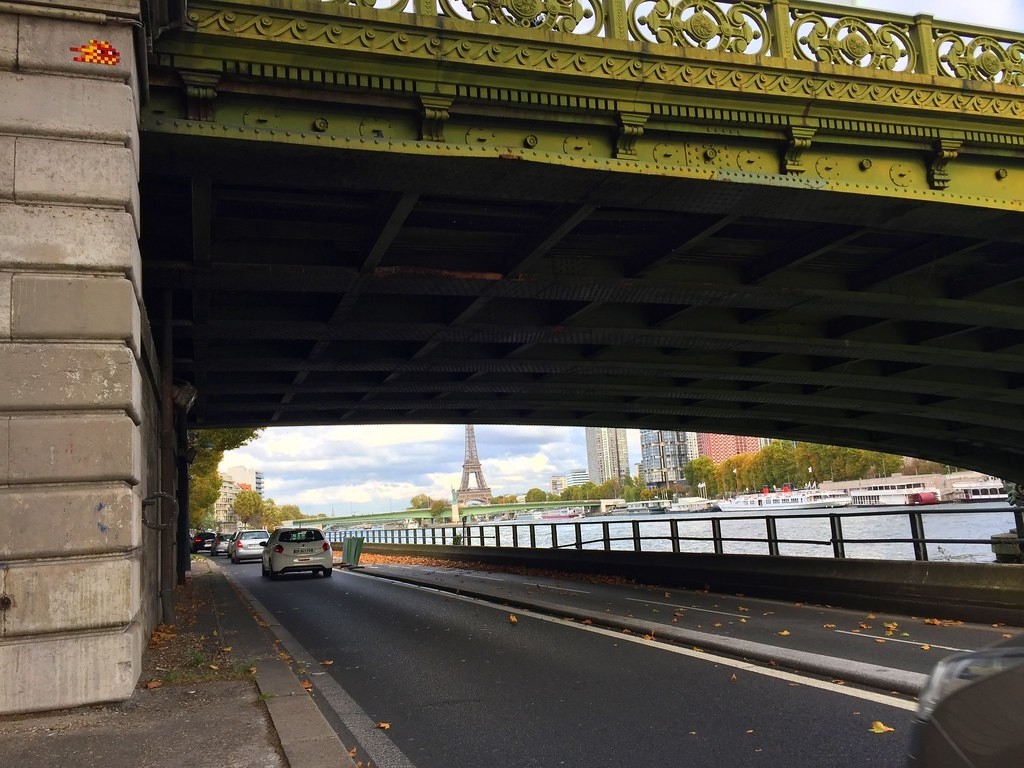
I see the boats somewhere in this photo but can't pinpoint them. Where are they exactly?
[493,507,587,520]
[611,482,956,515]
[955,476,1010,502]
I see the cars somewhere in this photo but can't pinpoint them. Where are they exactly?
[259,526,333,582]
[211,533,234,556]
[227,530,271,564]
[190,531,216,553]
